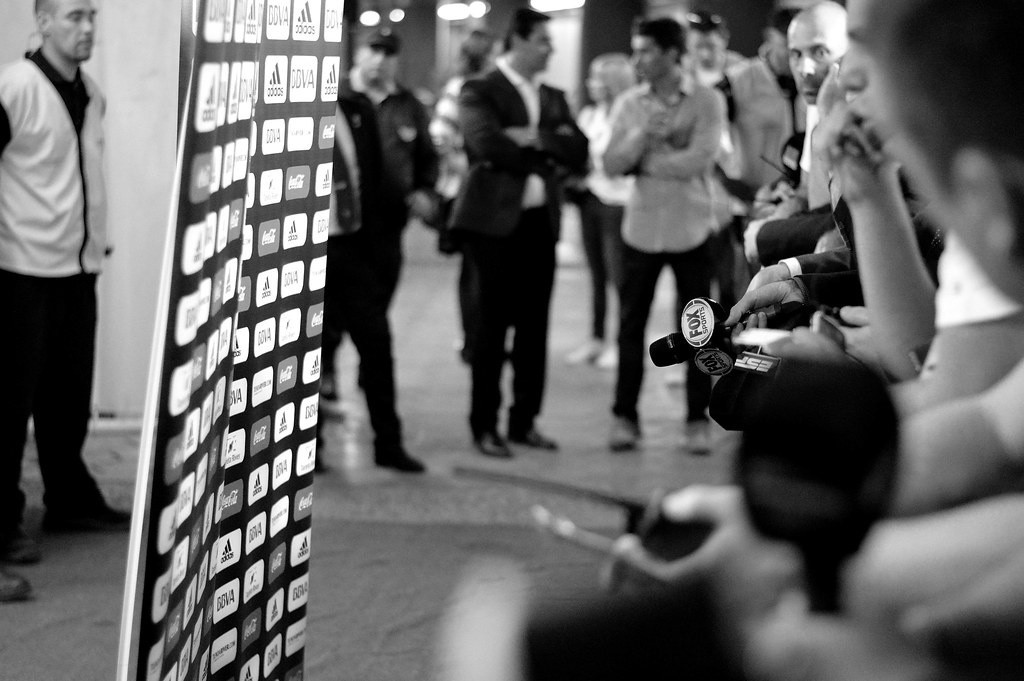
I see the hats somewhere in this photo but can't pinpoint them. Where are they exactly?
[363,30,403,49]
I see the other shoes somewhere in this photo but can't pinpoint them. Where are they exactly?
[374,441,426,476]
[683,414,714,451]
[608,410,638,447]
[595,346,618,368]
[567,338,602,362]
[0,529,39,567]
[41,507,131,534]
[0,570,32,602]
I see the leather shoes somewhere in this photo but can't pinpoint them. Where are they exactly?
[507,425,554,448]
[472,426,512,457]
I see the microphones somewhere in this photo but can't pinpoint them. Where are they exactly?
[650,296,806,376]
[709,344,781,432]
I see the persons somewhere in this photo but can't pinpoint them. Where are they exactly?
[0,0,133,604]
[316,0,1024,681]
